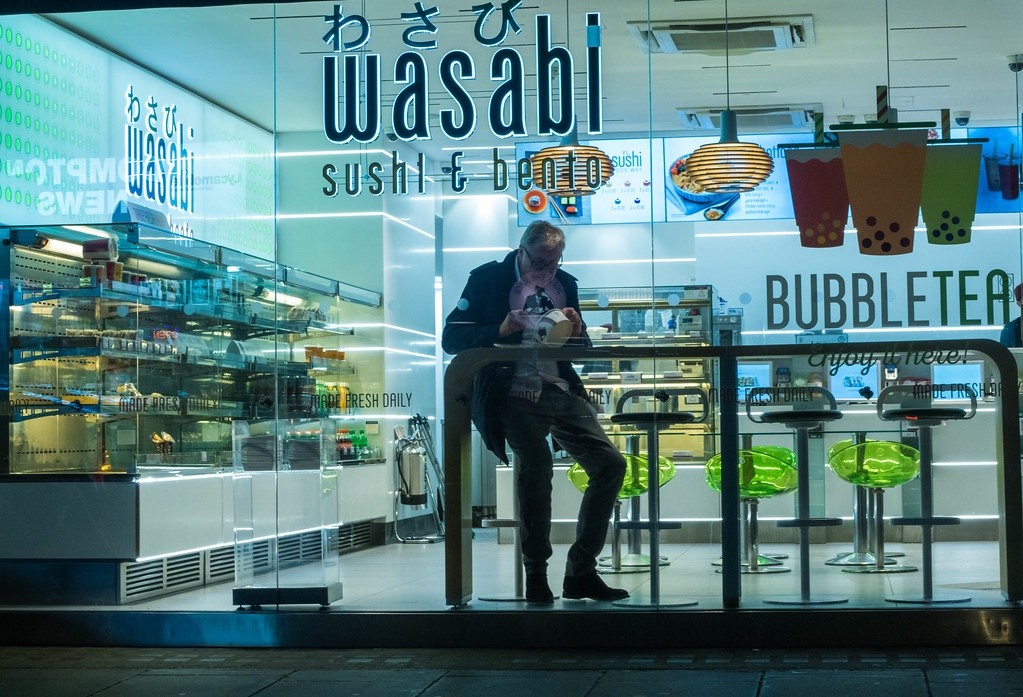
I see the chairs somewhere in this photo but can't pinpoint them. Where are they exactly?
[829,439,878,459]
[633,454,676,565]
[706,451,790,574]
[829,441,920,574]
[571,454,650,575]
[751,445,796,468]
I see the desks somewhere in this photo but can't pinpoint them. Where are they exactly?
[446,340,1023,606]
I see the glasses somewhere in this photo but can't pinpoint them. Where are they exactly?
[1015,294,1023,301]
[520,244,564,272]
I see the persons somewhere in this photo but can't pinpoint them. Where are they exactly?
[443,221,632,604]
[999,283,1023,348]
[522,284,555,316]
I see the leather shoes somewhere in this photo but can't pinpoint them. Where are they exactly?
[562,571,630,602]
[526,572,554,605]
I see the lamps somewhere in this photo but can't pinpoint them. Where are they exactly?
[684,1,775,193]
[531,0,615,197]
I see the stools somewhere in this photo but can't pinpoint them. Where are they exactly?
[876,384,977,603]
[746,386,848,604]
[612,387,709,608]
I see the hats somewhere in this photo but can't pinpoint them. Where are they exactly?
[1014,282,1022,296]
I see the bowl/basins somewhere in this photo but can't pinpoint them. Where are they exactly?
[532,307,574,348]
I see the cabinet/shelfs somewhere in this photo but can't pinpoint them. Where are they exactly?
[1,222,382,481]
[578,286,715,460]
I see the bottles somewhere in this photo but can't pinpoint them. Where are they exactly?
[82,236,149,290]
[286,426,381,462]
